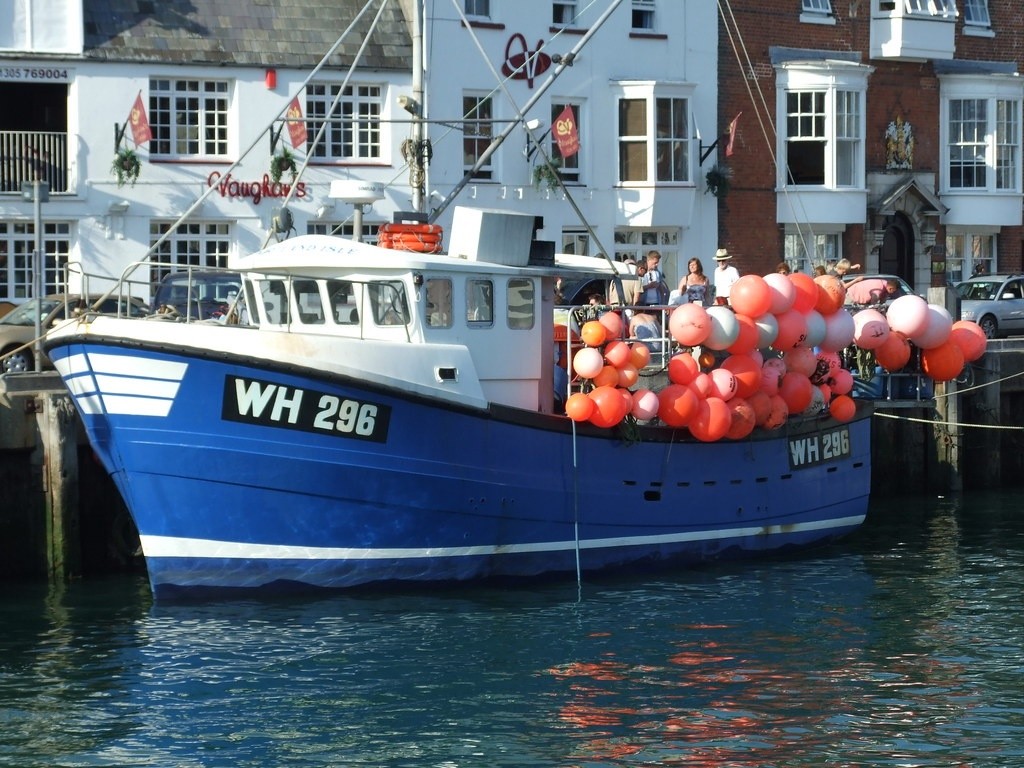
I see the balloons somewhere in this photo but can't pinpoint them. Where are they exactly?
[730,273,856,430]
[853,295,987,382]
[660,303,762,442]
[565,311,659,429]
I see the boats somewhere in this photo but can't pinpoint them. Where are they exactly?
[41,0,875,602]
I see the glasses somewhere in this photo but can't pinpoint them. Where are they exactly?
[717,260,726,262]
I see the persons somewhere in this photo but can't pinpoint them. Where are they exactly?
[757,258,863,289]
[970,264,985,276]
[588,294,606,321]
[629,300,664,365]
[610,250,667,306]
[678,257,715,305]
[843,278,901,311]
[712,249,740,305]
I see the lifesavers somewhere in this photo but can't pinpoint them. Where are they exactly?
[378,224,443,253]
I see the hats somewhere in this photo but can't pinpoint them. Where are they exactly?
[594,253,605,258]
[624,258,636,265]
[712,248,733,260]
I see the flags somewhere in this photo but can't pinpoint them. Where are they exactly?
[550,104,579,159]
[286,94,308,149]
[726,117,737,156]
[128,94,152,149]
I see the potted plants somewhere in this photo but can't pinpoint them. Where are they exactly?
[533,158,564,197]
[270,146,299,185]
[109,146,141,189]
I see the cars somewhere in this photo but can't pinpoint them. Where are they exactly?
[843,271,926,315]
[0,294,146,374]
[954,274,1023,339]
[146,269,245,322]
